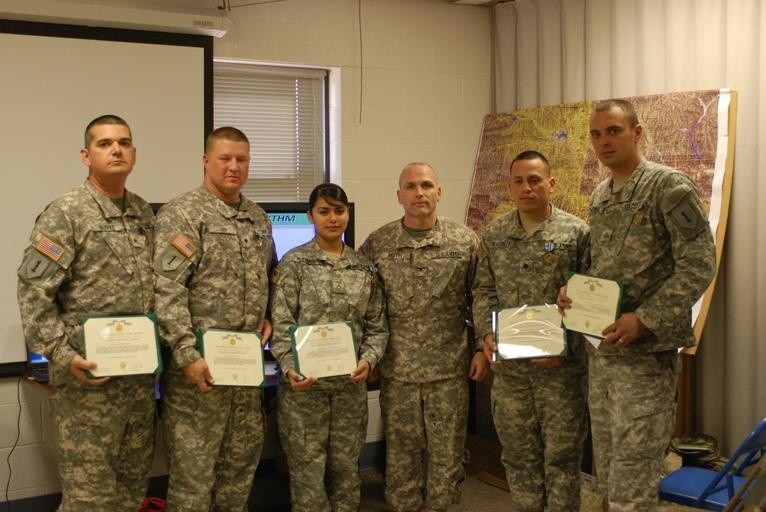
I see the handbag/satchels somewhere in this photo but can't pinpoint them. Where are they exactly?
[668,433,722,469]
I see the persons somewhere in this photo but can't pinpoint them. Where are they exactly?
[473,151,589,512]
[270,182,389,512]
[16,113,153,512]
[153,126,278,512]
[358,162,490,512]
[556,99,717,512]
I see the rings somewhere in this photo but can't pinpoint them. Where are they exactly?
[618,339,625,345]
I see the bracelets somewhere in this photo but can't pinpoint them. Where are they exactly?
[475,348,484,352]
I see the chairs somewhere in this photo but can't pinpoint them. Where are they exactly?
[658,418,766,511]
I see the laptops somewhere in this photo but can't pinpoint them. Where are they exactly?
[27,336,49,383]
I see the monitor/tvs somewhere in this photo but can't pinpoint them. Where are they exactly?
[150,203,355,361]
